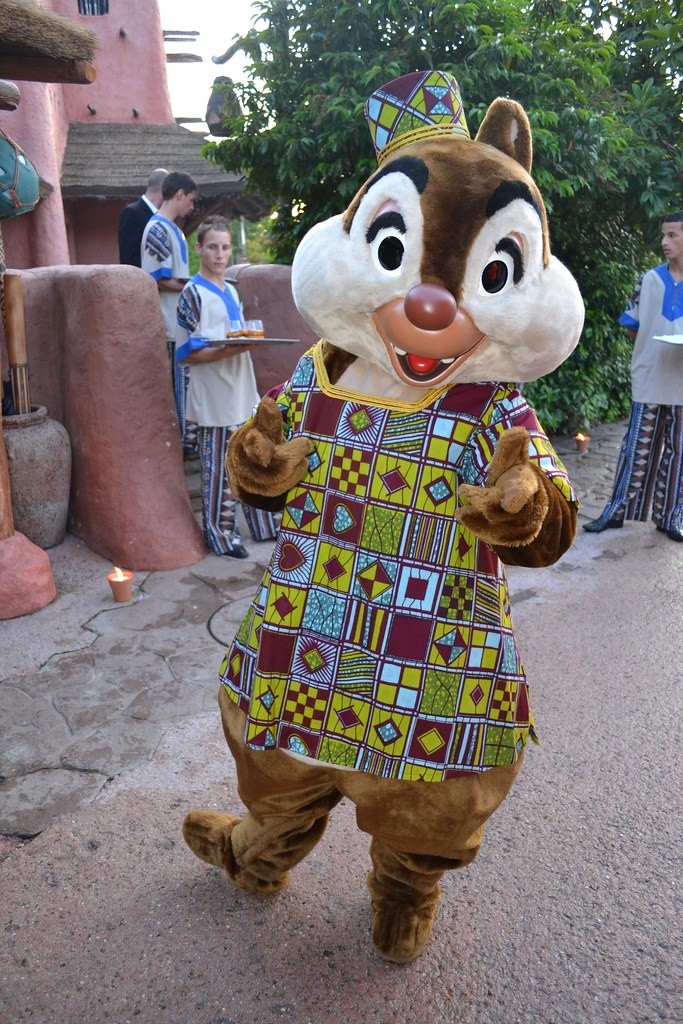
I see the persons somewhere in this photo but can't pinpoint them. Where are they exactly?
[138,172,201,459]
[582,212,683,543]
[120,169,171,268]
[176,215,284,560]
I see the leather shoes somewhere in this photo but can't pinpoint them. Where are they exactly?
[583,514,623,534]
[657,525,683,542]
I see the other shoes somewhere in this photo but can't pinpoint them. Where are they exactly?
[224,546,249,558]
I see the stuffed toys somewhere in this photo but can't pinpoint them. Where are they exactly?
[183,70,584,963]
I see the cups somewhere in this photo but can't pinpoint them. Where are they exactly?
[107,572,134,602]
[224,319,265,339]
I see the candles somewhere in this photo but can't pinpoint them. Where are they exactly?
[106,564,134,604]
[575,432,591,451]
